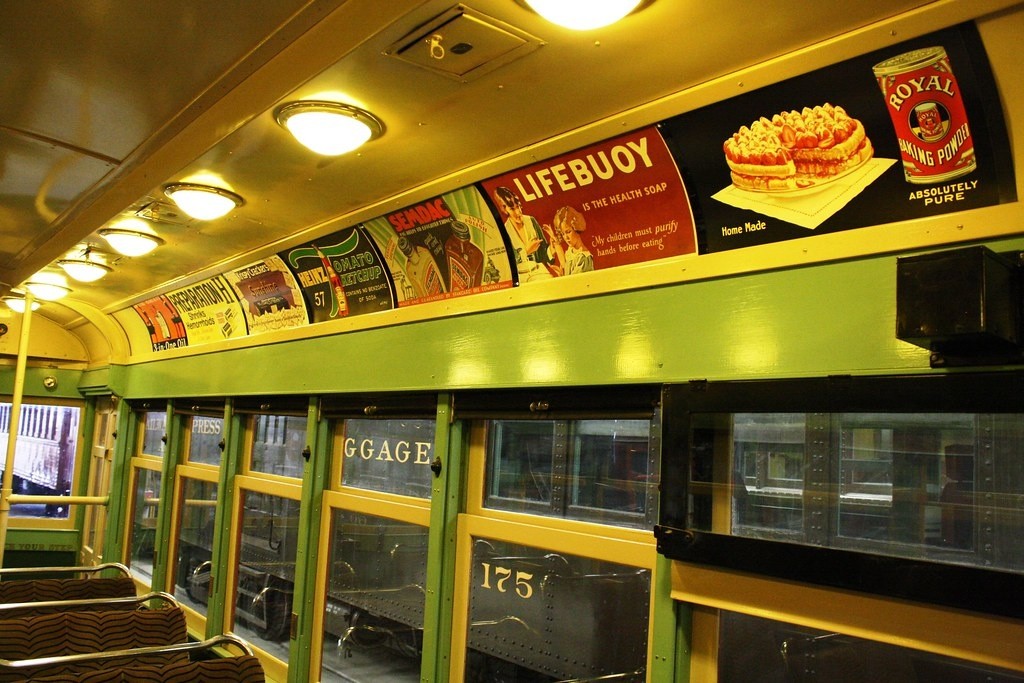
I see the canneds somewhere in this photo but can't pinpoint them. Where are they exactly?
[915,103,944,141]
[871,46,977,185]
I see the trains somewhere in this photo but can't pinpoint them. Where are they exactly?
[0,373,1024,683]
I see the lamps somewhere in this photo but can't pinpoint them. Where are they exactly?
[3,298,44,315]
[164,184,245,221]
[514,0,656,31]
[278,100,385,157]
[25,282,73,300]
[99,230,165,257]
[59,260,114,283]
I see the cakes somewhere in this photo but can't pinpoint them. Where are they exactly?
[724,102,873,193]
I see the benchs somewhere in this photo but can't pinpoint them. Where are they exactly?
[0,562,265,683]
